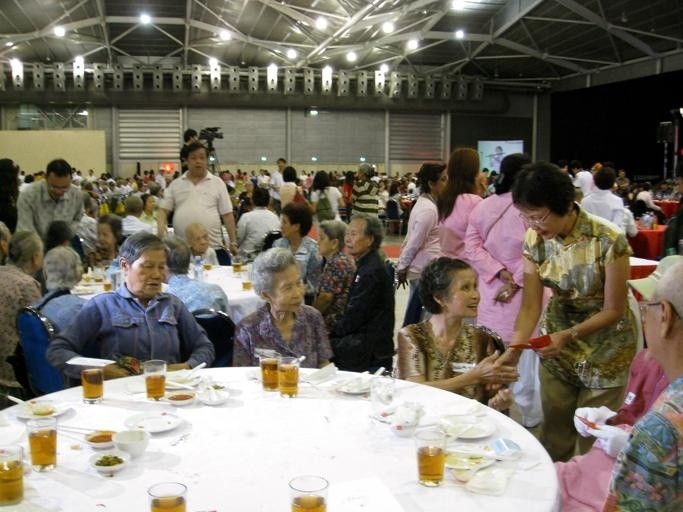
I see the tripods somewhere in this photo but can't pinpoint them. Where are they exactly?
[206,147,223,176]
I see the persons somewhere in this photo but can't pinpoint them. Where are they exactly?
[1,130,681,422]
[603,265,683,512]
[551,257,681,512]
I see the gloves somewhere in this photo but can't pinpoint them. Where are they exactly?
[573,406,617,437]
[587,424,628,457]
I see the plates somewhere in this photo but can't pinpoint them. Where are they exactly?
[123,406,180,434]
[441,412,496,440]
[164,363,230,405]
[11,400,71,417]
[335,371,424,428]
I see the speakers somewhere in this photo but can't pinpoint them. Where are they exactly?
[656,122,673,143]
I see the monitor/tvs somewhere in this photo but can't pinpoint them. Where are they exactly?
[476,139,524,178]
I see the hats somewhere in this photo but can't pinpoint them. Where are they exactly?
[626,255,683,296]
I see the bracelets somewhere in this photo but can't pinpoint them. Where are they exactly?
[228,240,239,248]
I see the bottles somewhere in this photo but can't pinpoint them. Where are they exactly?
[192,256,204,280]
[106,256,120,291]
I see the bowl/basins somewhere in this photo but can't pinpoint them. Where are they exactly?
[83,428,149,471]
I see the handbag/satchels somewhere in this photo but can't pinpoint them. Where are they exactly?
[6,343,29,388]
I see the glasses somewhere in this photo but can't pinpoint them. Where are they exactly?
[519,209,552,224]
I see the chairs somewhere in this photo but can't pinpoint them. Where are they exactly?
[189,307,236,369]
[13,306,72,396]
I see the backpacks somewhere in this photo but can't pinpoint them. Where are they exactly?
[315,191,335,221]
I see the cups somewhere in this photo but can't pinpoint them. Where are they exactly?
[26,415,59,470]
[79,363,103,402]
[259,351,300,400]
[142,357,166,400]
[285,473,329,511]
[229,254,250,292]
[0,445,22,508]
[101,268,114,290]
[414,428,445,487]
[148,480,187,511]
